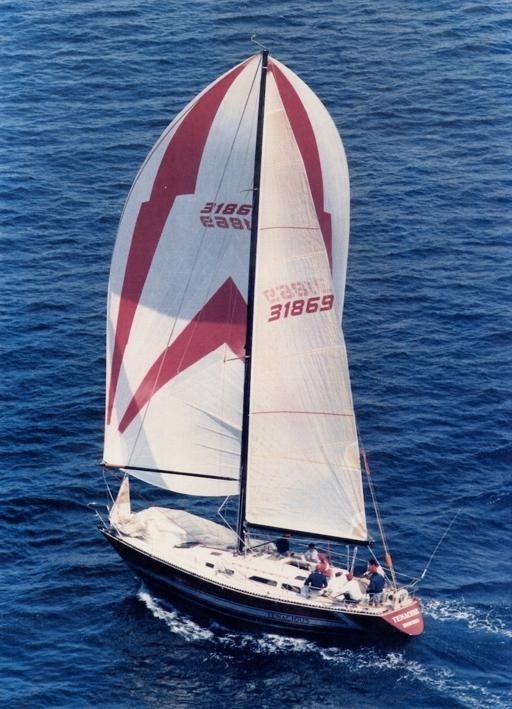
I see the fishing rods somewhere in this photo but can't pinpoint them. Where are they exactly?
[418,506,463,581]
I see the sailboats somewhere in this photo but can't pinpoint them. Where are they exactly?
[89,44,431,649]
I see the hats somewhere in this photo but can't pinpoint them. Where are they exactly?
[316,553,331,576]
[346,573,353,580]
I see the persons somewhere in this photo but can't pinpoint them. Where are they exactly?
[275,532,292,558]
[304,542,386,607]
